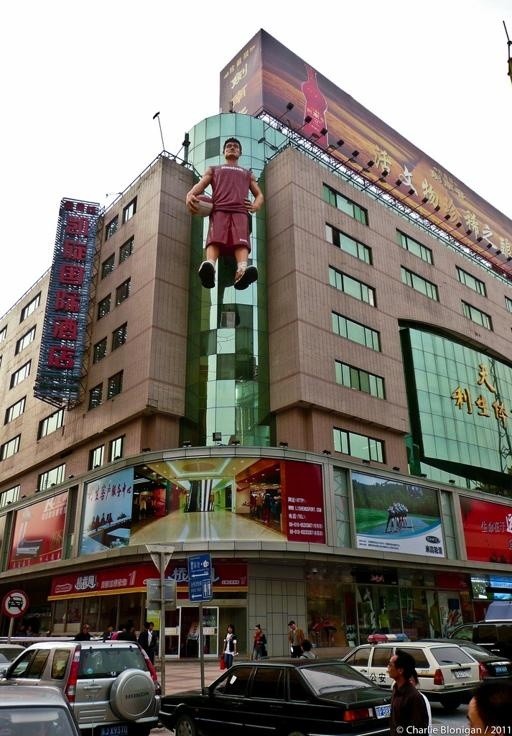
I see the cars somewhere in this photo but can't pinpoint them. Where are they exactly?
[160,657,393,736]
[338,639,512,709]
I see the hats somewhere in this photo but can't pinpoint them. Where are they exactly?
[288,621,295,626]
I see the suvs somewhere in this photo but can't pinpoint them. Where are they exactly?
[0,639,163,736]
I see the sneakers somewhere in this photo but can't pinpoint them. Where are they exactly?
[199,261,215,288]
[234,266,258,290]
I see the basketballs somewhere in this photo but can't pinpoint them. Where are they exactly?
[193,193,214,217]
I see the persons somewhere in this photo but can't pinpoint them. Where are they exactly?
[467,682,512,736]
[251,625,268,660]
[408,670,432,736]
[245,489,281,524]
[222,624,238,670]
[447,600,458,626]
[378,596,391,633]
[287,621,305,658]
[13,622,47,647]
[75,623,91,640]
[186,138,264,291]
[385,502,410,533]
[299,640,315,660]
[387,650,429,736]
[101,617,159,666]
[218,668,250,694]
[430,592,442,638]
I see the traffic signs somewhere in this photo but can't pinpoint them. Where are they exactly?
[188,553,213,602]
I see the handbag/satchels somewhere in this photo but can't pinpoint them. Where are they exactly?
[221,656,226,669]
